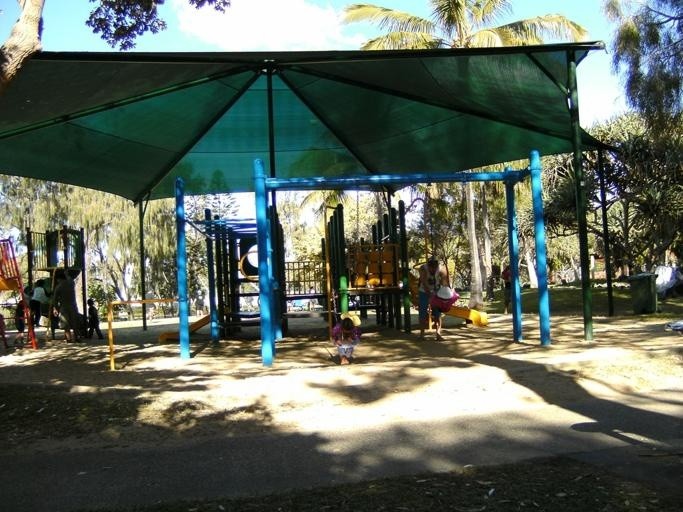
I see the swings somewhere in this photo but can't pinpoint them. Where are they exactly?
[424,180,467,314]
[322,181,360,348]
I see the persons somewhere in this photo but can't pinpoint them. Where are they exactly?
[332,318,361,365]
[502,266,511,314]
[53,272,83,343]
[419,260,458,341]
[88,299,104,339]
[16,279,51,343]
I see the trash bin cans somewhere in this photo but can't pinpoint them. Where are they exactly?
[626,273,658,314]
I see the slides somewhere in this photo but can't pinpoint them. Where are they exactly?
[397,267,489,327]
[157,304,229,344]
